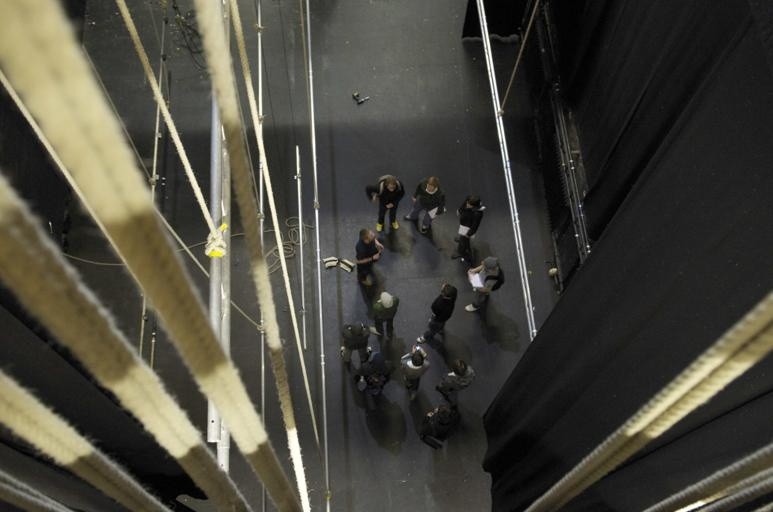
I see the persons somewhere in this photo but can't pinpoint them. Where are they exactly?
[417,403,460,451]
[403,175,446,234]
[364,173,404,232]
[399,343,432,403]
[355,229,385,287]
[464,256,504,312]
[367,290,400,338]
[416,283,458,344]
[354,349,395,397]
[340,321,369,363]
[434,359,476,405]
[455,193,485,266]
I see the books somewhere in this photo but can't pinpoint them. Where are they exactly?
[458,225,475,239]
[468,272,483,288]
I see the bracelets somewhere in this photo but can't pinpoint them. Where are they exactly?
[371,255,373,262]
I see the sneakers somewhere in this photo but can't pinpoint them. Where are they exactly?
[392,219,399,229]
[376,223,383,232]
[404,215,419,221]
[416,336,426,343]
[369,326,384,336]
[464,304,480,312]
[422,224,428,234]
[340,346,346,357]
[361,347,372,363]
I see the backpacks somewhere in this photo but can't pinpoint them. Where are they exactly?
[484,267,505,291]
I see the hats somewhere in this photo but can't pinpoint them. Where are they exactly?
[381,291,393,309]
